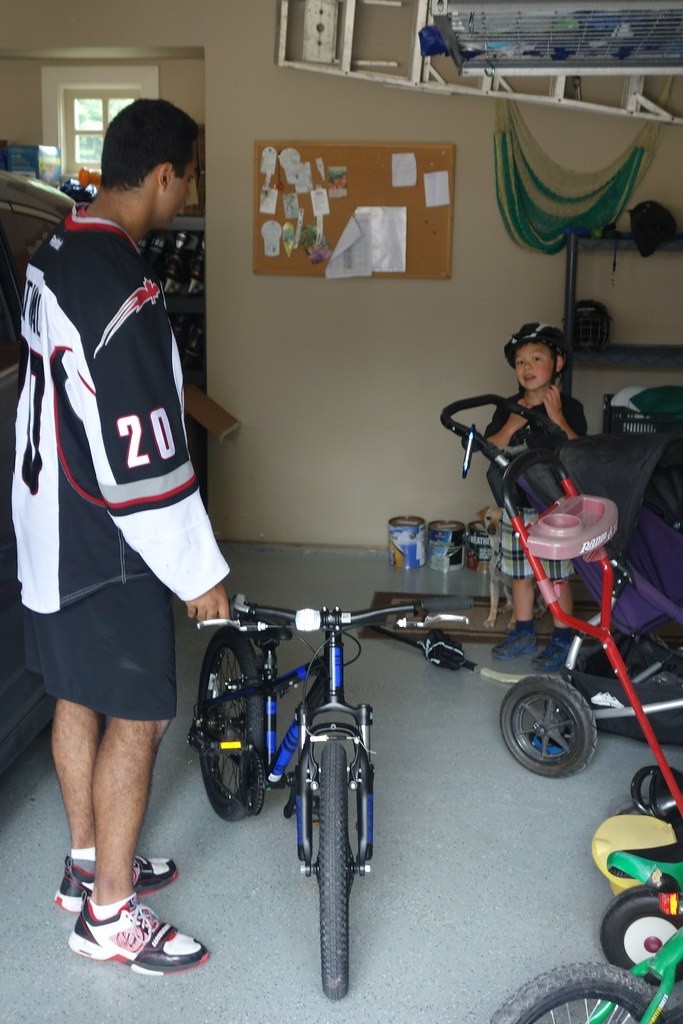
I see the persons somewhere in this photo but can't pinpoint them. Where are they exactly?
[10,96,232,975]
[482,322,587,671]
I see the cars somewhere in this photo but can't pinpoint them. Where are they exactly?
[0,173,73,766]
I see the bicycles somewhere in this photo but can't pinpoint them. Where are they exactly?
[188,592,472,1002]
[487,844,683,1024]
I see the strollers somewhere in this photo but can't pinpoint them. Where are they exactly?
[438,395,683,779]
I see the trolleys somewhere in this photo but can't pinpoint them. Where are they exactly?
[438,391,683,989]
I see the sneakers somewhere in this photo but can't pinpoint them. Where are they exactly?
[492,629,536,659]
[54,856,179,913]
[68,890,209,974]
[533,635,574,669]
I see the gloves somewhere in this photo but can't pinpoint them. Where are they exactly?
[424,629,464,670]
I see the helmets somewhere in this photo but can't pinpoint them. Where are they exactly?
[562,298,613,354]
[504,322,568,374]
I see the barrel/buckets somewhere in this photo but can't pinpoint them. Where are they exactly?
[426,520,466,572]
[388,516,426,569]
[466,521,491,572]
